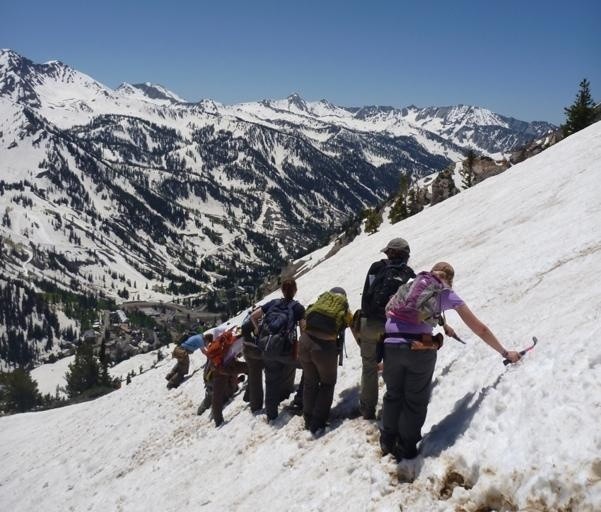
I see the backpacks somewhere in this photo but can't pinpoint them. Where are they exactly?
[206,330,235,370]
[255,298,299,363]
[383,271,453,328]
[363,258,409,323]
[300,291,348,366]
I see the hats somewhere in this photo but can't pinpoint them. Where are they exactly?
[379,238,409,254]
[429,262,453,275]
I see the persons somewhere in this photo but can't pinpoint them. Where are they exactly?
[359,238,455,419]
[166,334,219,388]
[377,262,522,460]
[197,279,353,439]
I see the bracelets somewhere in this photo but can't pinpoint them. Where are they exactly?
[502,349,508,357]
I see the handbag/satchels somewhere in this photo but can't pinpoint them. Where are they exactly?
[171,346,186,362]
